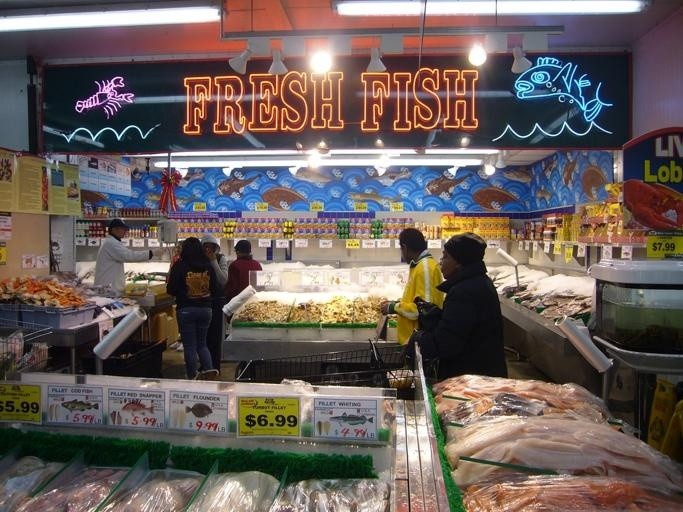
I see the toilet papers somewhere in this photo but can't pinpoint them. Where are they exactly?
[223,286,256,315]
[497,248,518,268]
[554,313,615,374]
[95,306,145,360]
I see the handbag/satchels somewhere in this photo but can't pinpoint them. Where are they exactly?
[406,297,443,379]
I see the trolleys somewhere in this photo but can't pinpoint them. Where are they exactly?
[0,318,54,381]
[234,328,440,401]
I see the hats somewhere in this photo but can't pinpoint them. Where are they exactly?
[234,240,251,253]
[110,219,130,227]
[445,232,487,265]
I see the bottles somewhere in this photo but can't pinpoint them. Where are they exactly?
[282,219,295,241]
[335,219,350,240]
[372,219,383,239]
[74,200,151,240]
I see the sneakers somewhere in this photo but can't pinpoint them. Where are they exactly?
[169,341,184,351]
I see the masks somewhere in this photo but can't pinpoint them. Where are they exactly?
[401,251,411,263]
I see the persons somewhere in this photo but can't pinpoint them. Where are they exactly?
[94,218,164,295]
[380,228,445,373]
[221,240,262,382]
[200,235,227,373]
[167,236,217,381]
[414,233,507,382]
[163,240,185,352]
